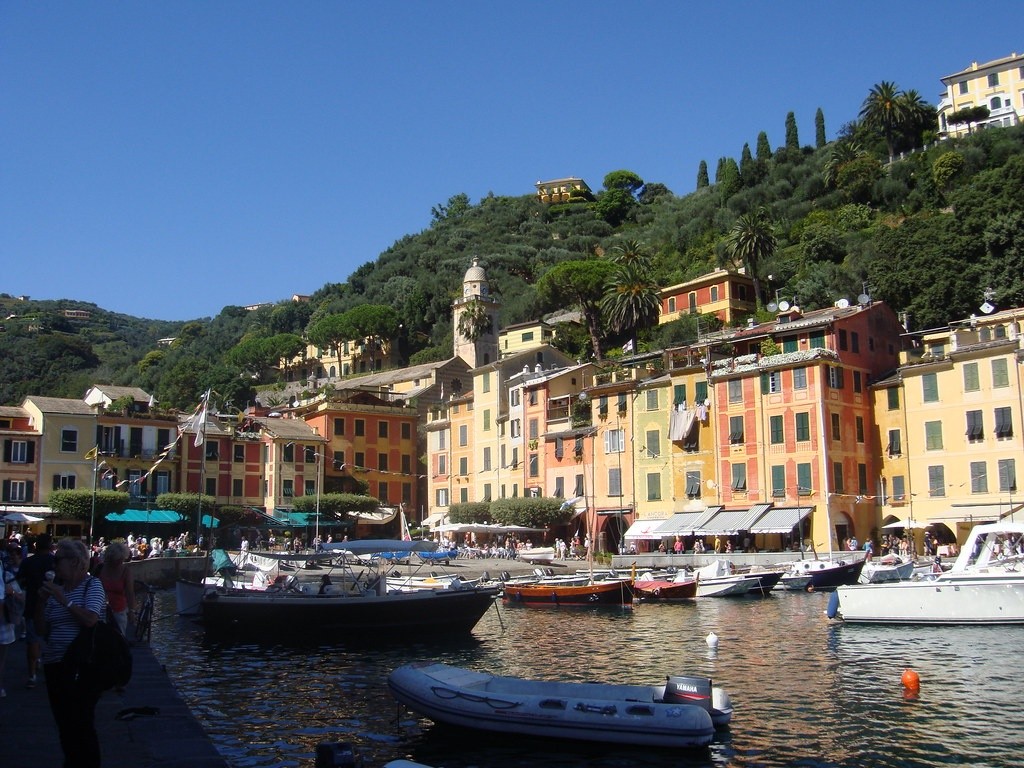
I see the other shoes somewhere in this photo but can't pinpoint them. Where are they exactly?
[21,632,27,639]
[24,673,36,687]
[37,658,40,669]
[0,688,6,697]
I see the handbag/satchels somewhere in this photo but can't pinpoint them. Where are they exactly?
[66,620,134,690]
[6,593,25,626]
[0,623,16,644]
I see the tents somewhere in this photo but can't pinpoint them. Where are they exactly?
[429,521,550,545]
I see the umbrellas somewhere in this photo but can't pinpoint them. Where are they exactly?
[881,517,931,556]
[4,514,45,532]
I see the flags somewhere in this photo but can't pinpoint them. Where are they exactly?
[192,400,207,448]
[84,391,208,489]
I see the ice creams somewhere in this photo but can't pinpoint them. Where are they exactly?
[44,569,55,584]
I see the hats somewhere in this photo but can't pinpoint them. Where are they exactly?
[715,535,719,538]
[555,538,559,541]
[924,531,930,535]
[559,539,563,541]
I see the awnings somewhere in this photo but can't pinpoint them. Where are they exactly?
[421,513,447,525]
[653,502,815,536]
[0,505,60,513]
[623,518,668,540]
[273,507,398,527]
[561,507,586,526]
[102,506,220,528]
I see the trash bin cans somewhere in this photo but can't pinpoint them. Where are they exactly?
[261,540,266,549]
[165,549,176,557]
[178,550,190,557]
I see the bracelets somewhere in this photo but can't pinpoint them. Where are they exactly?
[64,601,74,611]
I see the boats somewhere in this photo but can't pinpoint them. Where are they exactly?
[172,539,510,628]
[867,554,911,582]
[505,568,699,608]
[389,662,733,748]
[314,741,433,768]
[700,550,867,597]
[833,523,1024,627]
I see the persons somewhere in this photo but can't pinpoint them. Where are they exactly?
[864,537,876,556]
[38,538,108,768]
[239,536,249,569]
[91,536,129,638]
[888,534,908,556]
[785,540,812,551]
[743,535,758,553]
[0,527,59,699]
[618,541,637,555]
[659,538,706,553]
[127,532,205,559]
[265,576,285,593]
[842,534,858,551]
[715,536,721,553]
[724,540,732,553]
[555,535,589,562]
[923,532,938,556]
[318,575,332,594]
[423,536,532,558]
[255,535,348,551]
